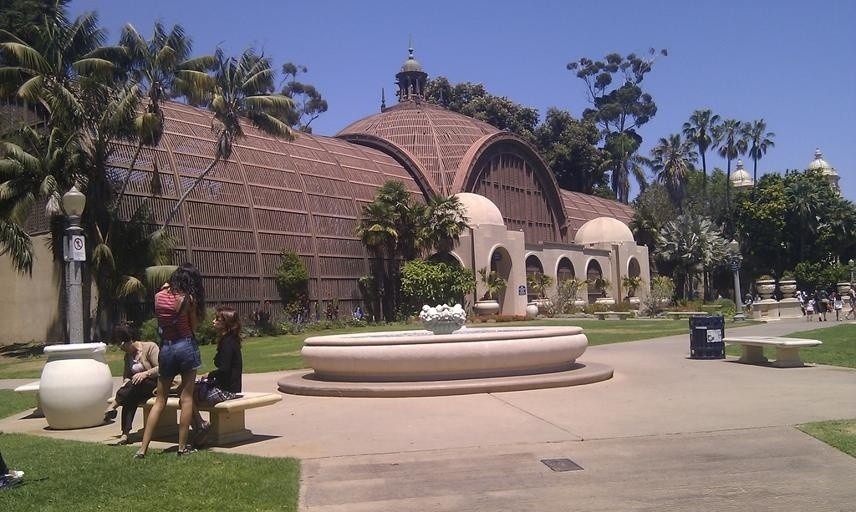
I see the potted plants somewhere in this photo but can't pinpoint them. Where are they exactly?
[778,270,797,297]
[472,266,677,316]
[836,271,852,296]
[755,274,776,298]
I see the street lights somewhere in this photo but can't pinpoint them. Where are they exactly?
[60,185,86,345]
[730,239,743,315]
[848,258,854,286]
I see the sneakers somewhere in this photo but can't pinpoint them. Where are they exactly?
[103,408,118,422]
[134,451,145,458]
[0,470,23,488]
[107,436,128,446]
[175,423,212,459]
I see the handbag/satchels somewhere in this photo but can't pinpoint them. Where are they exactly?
[153,374,182,396]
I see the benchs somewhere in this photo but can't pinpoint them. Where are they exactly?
[666,311,709,320]
[592,311,631,321]
[722,336,824,368]
[701,304,723,315]
[106,391,283,446]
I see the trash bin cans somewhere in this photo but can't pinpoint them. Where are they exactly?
[687,315,726,360]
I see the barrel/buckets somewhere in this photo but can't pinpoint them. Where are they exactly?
[689,317,725,358]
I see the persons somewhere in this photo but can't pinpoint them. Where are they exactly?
[0,452,25,487]
[104,324,158,445]
[176,303,243,446]
[745,283,856,324]
[132,262,207,461]
